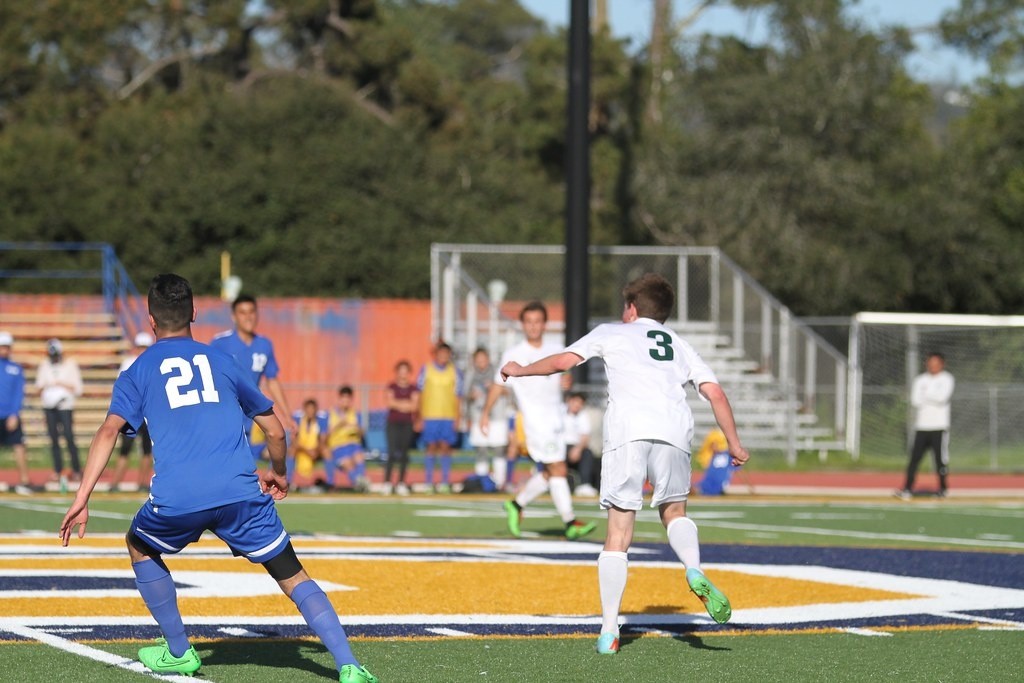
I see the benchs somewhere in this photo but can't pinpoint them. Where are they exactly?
[429,319,846,465]
[0,311,139,452]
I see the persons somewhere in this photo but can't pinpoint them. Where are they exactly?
[414,344,463,495]
[462,346,508,488]
[385,361,418,495]
[104,331,151,493]
[500,274,748,653]
[480,303,596,541]
[211,297,298,436]
[697,430,727,466]
[893,352,954,501]
[329,386,369,493]
[59,272,377,682]
[0,332,42,495]
[564,394,599,489]
[250,423,273,469]
[504,414,527,491]
[290,400,335,491]
[35,338,83,492]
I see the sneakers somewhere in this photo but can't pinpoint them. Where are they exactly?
[1,483,9,490]
[66,482,79,491]
[895,491,911,501]
[502,500,521,538]
[293,479,603,497]
[339,665,377,683]
[138,637,202,677]
[565,520,597,540]
[598,634,620,655]
[685,569,732,625]
[46,480,61,491]
[16,484,36,494]
[933,488,950,498]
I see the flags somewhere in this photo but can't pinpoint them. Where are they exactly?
[698,453,734,492]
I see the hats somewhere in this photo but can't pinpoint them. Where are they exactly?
[135,331,153,347]
[1,332,12,345]
[46,339,63,355]
